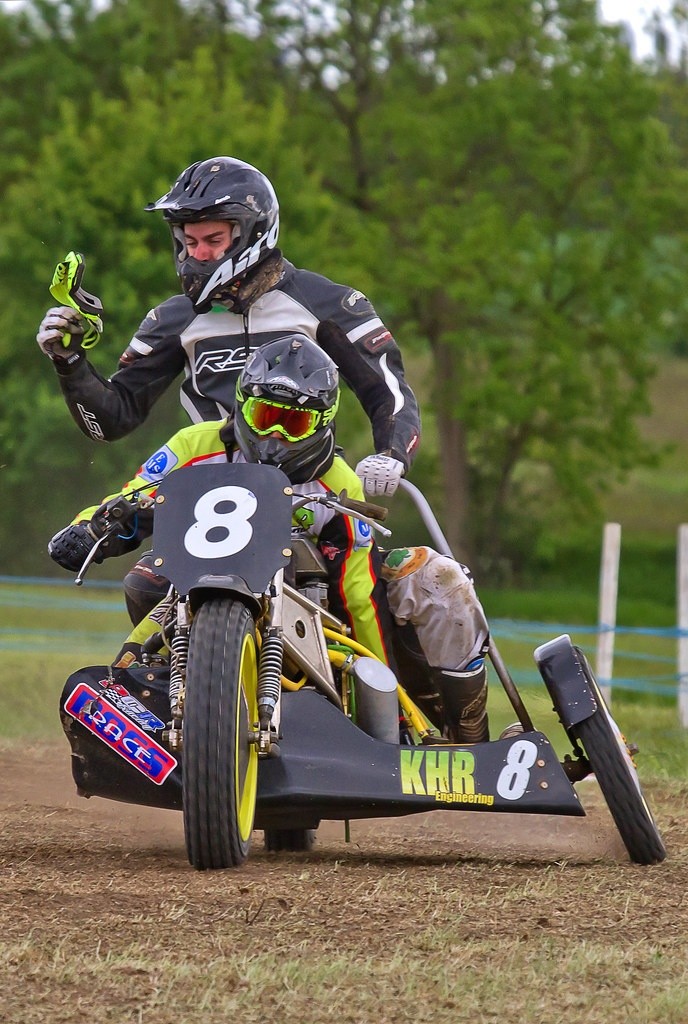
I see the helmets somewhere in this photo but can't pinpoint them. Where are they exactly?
[234,334,341,476]
[142,155,280,308]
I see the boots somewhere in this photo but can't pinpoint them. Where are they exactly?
[430,662,490,743]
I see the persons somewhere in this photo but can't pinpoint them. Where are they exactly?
[36,156,421,498]
[48,334,401,682]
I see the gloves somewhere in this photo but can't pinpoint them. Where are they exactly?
[35,306,87,374]
[47,524,104,572]
[355,455,404,497]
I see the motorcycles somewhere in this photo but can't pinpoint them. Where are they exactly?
[58,463,666,869]
